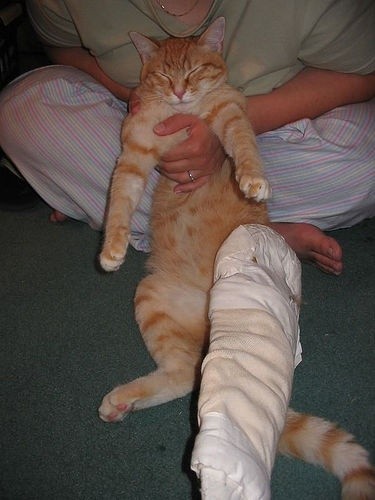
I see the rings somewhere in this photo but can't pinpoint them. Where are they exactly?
[186,169,198,184]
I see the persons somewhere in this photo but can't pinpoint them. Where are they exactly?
[0,0,375,278]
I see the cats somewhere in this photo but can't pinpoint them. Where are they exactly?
[95,17,375,500]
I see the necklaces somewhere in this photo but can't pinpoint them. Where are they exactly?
[156,0,200,17]
